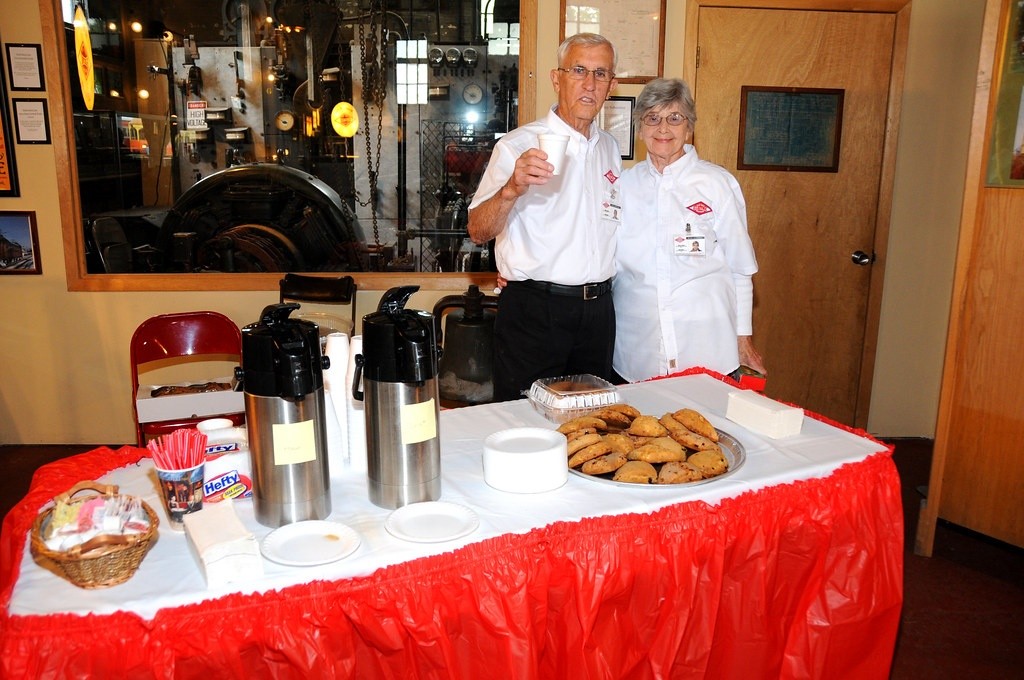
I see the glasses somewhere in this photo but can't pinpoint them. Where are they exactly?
[558,66,616,83]
[640,112,689,126]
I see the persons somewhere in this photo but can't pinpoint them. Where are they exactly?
[602,76,769,388]
[612,210,619,220]
[467,36,623,404]
[690,241,701,252]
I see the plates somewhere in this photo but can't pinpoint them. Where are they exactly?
[261,520,361,565]
[569,428,746,488]
[384,501,479,542]
[482,428,568,493]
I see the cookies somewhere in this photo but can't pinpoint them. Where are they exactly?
[557,402,728,484]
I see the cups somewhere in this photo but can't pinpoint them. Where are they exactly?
[196,418,233,439]
[155,458,206,531]
[322,332,368,475]
[537,134,570,175]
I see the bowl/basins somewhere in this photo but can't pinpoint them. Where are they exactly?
[521,374,621,424]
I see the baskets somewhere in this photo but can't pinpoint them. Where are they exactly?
[29,481,161,590]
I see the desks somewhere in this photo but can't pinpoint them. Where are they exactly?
[0,366,904,678]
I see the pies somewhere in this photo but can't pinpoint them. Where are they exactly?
[151,382,230,398]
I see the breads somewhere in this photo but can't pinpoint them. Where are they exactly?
[544,381,616,424]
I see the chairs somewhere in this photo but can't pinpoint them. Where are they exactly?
[278,273,357,337]
[91,217,133,274]
[130,311,245,449]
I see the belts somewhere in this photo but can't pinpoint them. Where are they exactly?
[515,279,612,300]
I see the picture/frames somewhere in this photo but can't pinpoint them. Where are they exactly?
[0,41,20,198]
[0,209,42,276]
